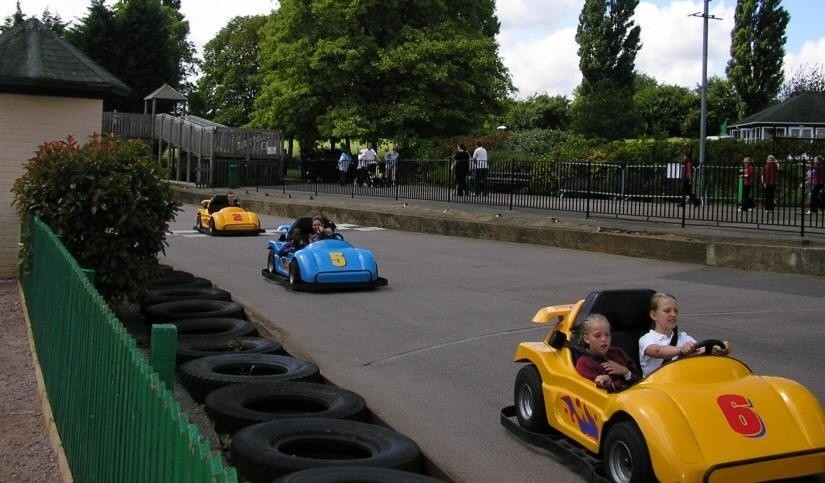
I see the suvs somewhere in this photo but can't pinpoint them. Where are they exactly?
[706,134,736,140]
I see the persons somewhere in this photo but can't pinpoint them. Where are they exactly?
[639,293,730,379]
[308,144,324,183]
[451,142,489,196]
[336,142,400,188]
[224,191,241,208]
[740,153,825,216]
[676,153,701,208]
[278,226,309,257]
[576,314,642,392]
[308,215,333,243]
[283,149,288,178]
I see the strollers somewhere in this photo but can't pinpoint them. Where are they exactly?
[362,158,390,188]
